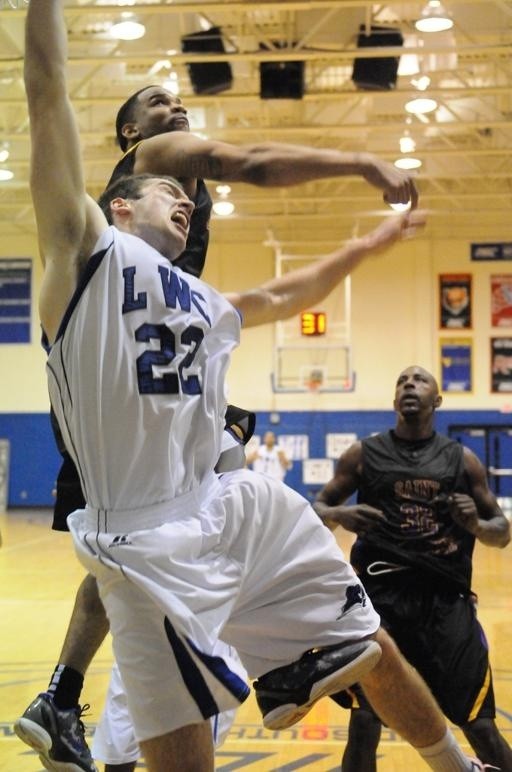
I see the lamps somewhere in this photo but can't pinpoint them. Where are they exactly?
[109,0,455,215]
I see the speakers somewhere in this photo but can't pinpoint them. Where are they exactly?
[181,27,232,96]
[258,41,303,99]
[350,24,403,91]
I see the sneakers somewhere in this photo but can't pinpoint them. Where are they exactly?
[14,691,98,772]
[253,640,382,732]
